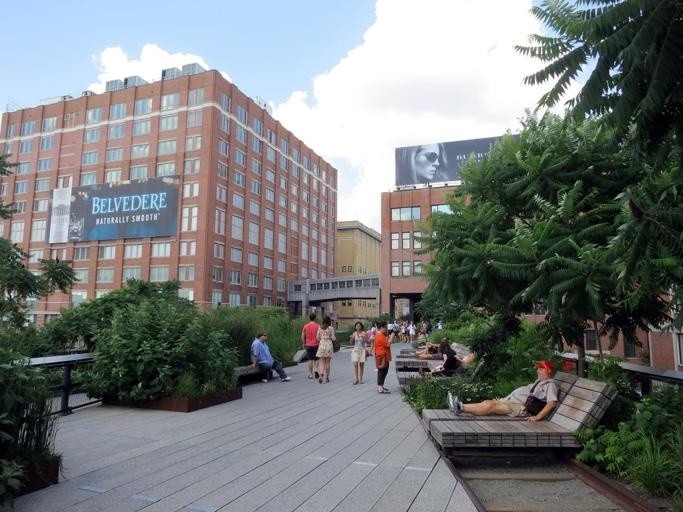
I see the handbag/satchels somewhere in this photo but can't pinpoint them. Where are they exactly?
[376,355,386,368]
[332,341,340,353]
[525,395,547,415]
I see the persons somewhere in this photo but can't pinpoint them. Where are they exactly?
[446,360,558,423]
[248,330,291,385]
[396,141,450,184]
[299,313,477,395]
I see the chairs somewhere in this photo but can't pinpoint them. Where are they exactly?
[419,368,579,433]
[426,375,620,461]
[394,341,480,389]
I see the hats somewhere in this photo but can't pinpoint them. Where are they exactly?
[533,360,553,377]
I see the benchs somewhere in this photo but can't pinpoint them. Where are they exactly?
[230,360,275,386]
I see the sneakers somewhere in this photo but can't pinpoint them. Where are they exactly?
[447,391,463,416]
